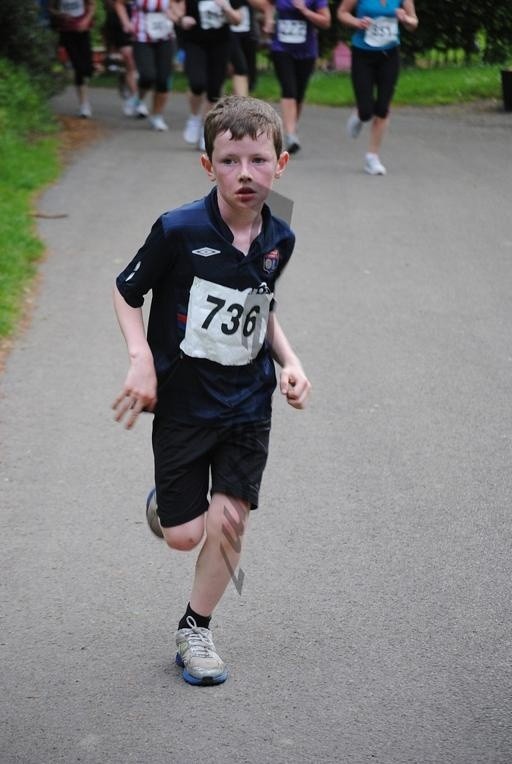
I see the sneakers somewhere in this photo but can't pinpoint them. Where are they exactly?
[77,99,93,117]
[143,485,164,544]
[183,112,202,145]
[120,93,171,133]
[360,150,386,178]
[173,626,231,688]
[283,133,302,153]
[346,105,365,140]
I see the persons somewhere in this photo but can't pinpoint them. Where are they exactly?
[110,95,312,687]
